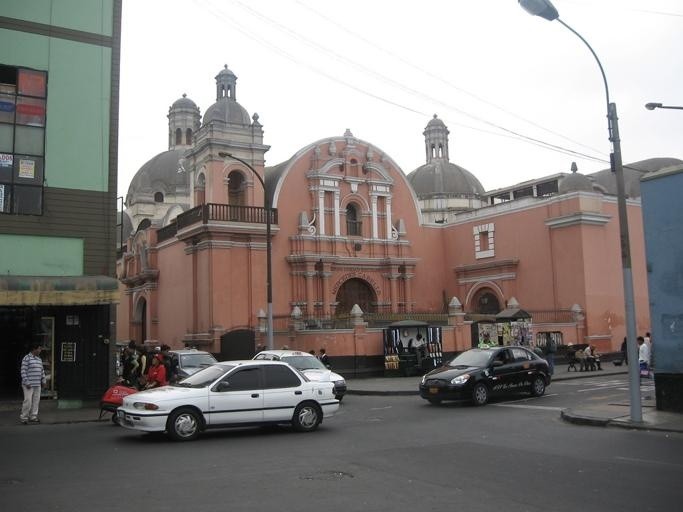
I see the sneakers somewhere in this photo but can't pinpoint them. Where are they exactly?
[29,418,39,422]
[18,419,27,424]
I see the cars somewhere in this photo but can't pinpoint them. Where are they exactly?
[251,349,347,402]
[165,349,219,384]
[115,359,340,443]
[417,344,552,405]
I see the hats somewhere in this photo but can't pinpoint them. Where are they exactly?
[153,353,163,361]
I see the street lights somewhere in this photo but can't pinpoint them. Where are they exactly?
[514,1,645,424]
[216,152,274,351]
[644,102,683,110]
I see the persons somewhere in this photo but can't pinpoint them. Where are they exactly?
[574,346,587,372]
[479,331,495,346]
[410,334,429,362]
[644,333,650,352]
[20,343,46,424]
[621,338,628,365]
[584,344,600,371]
[121,340,177,390]
[309,351,320,360]
[638,336,653,386]
[319,350,331,369]
[546,331,554,375]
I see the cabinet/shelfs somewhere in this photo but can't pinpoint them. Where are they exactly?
[35,316,55,398]
